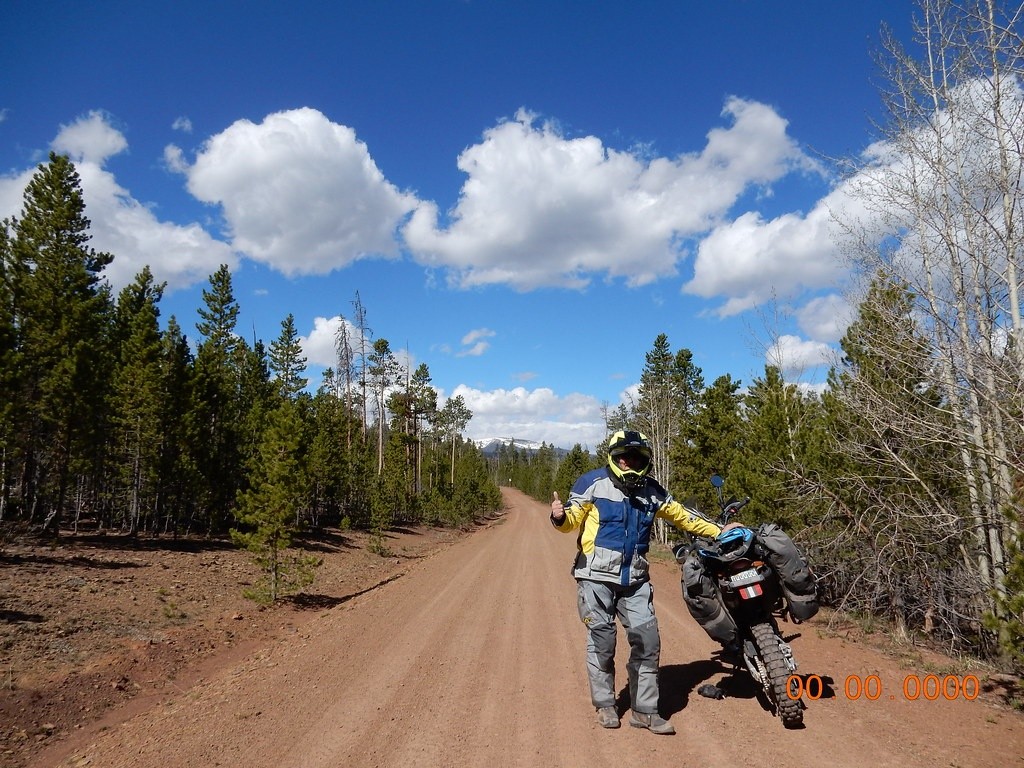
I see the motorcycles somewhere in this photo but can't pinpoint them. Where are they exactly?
[665,474,808,728]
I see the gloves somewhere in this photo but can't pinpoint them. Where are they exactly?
[698,685,727,700]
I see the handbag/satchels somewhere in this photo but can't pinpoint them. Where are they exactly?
[753,523,819,625]
[681,557,739,643]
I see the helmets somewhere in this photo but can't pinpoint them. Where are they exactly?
[608,430,654,490]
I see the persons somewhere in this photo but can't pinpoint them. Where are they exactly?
[550,430,745,734]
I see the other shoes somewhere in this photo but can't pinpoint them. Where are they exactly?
[630,709,674,734]
[597,705,620,727]
